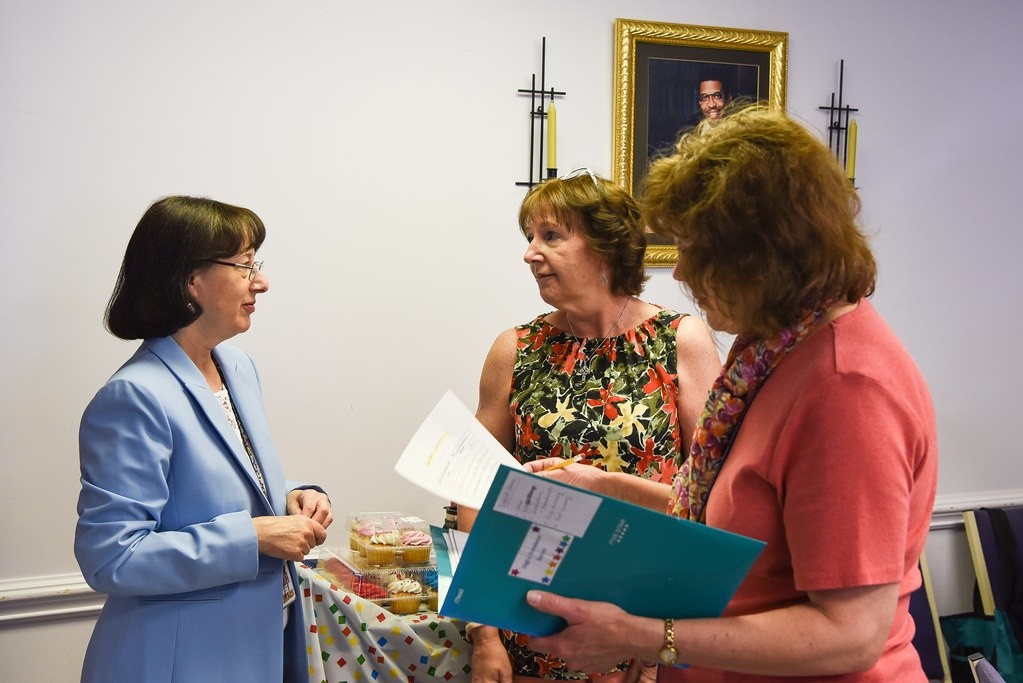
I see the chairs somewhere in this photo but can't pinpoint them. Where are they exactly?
[909,504,1023,683]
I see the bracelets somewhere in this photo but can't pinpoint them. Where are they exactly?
[465,622,483,643]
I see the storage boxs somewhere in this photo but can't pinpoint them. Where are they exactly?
[316,546,438,614]
[348,511,433,565]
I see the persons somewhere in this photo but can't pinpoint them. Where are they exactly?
[457,166,723,683]
[74,194,334,683]
[697,71,734,119]
[523,96,939,683]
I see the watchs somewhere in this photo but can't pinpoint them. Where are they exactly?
[659,618,679,666]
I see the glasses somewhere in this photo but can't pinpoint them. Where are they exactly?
[700,91,725,103]
[208,259,265,281]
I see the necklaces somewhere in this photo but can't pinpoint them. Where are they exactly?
[566,295,630,382]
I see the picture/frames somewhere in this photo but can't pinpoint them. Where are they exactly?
[616,20,789,269]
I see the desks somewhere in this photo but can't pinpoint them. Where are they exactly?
[292,561,473,683]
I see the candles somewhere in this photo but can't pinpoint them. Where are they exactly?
[846,118,858,176]
[547,100,557,166]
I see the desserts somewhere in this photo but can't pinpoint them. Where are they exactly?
[324,515,438,615]
